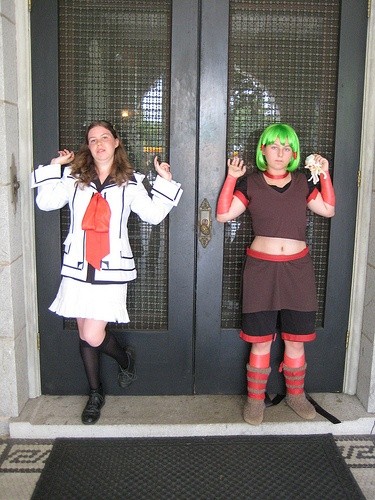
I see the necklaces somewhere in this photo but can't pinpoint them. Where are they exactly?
[263,169,289,180]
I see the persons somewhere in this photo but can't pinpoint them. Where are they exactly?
[29,120,183,424]
[216,123,336,424]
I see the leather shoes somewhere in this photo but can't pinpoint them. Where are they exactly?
[82,387,106,424]
[118,347,137,391]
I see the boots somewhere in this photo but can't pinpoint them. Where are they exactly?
[282,362,316,420]
[243,363,272,426]
